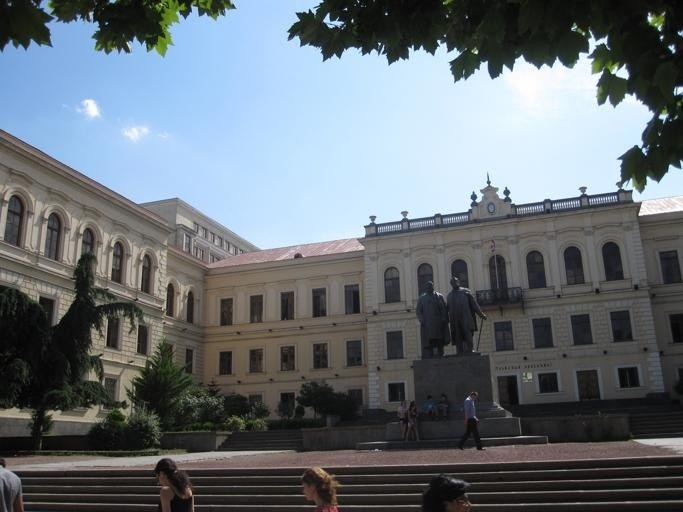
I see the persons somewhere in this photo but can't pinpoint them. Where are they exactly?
[447,276,488,354]
[154,458,195,512]
[457,390,487,450]
[395,391,451,441]
[301,466,341,512]
[0,458,25,512]
[415,281,451,356]
[422,473,473,512]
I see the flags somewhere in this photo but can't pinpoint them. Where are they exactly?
[488,238,497,252]
